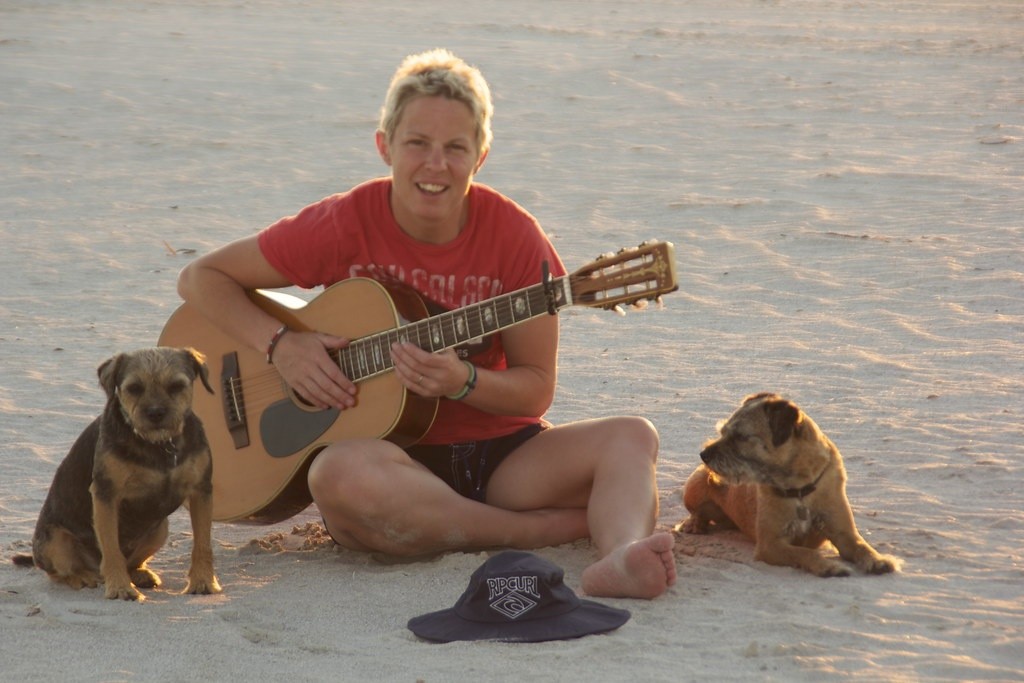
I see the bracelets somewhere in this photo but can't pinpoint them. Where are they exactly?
[266,326,288,364]
[445,359,476,401]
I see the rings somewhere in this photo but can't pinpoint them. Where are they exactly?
[417,376,424,384]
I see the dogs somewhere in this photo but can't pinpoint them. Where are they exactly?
[674,392,902,577]
[13,346,222,602]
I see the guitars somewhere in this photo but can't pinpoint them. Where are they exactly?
[154,236,681,527]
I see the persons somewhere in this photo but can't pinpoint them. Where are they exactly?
[177,45,678,601]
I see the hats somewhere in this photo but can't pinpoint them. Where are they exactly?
[408,551,632,642]
